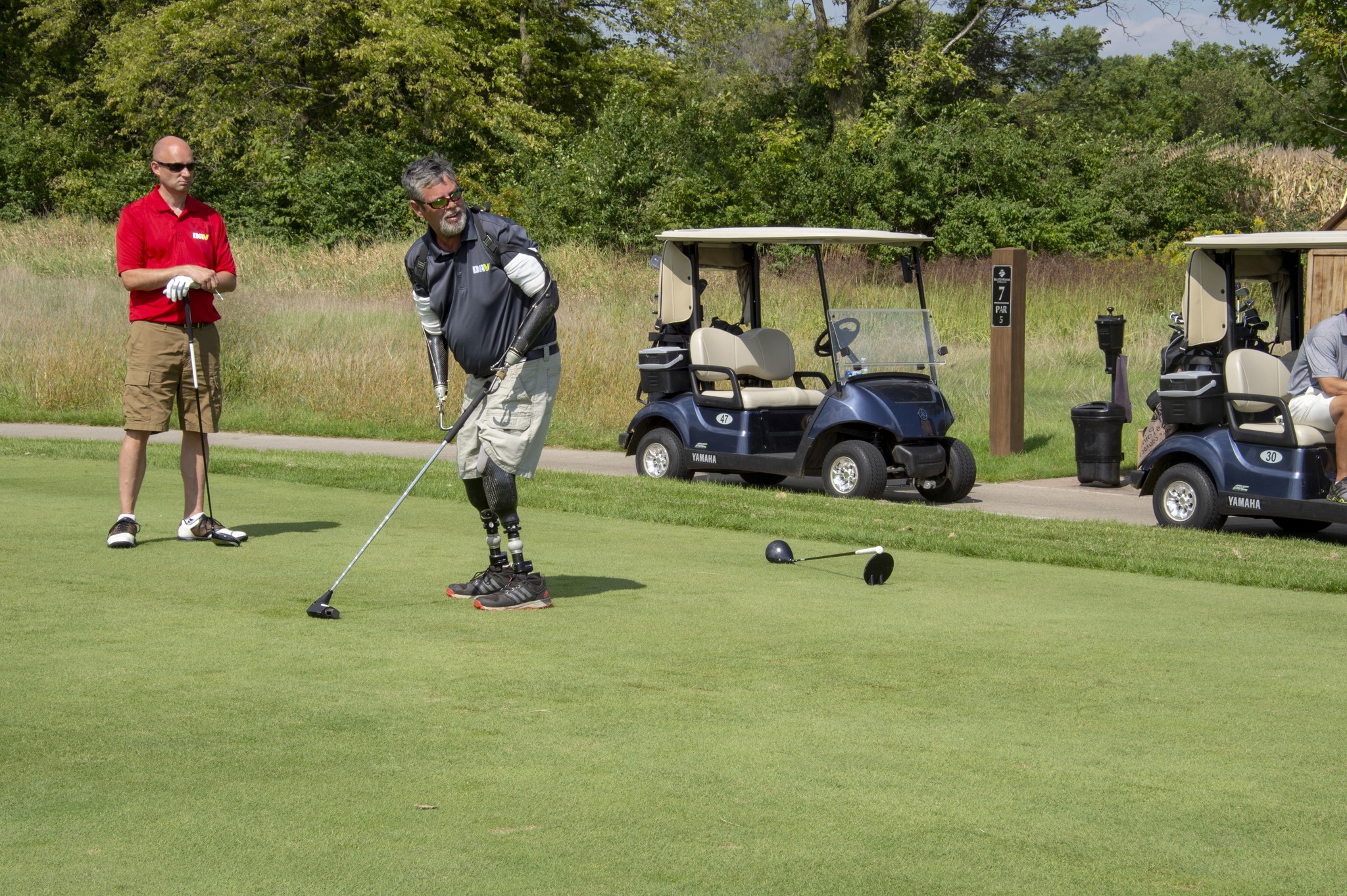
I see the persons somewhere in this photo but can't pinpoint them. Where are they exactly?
[1287,308,1347,506]
[401,158,561,611]
[107,136,247,545]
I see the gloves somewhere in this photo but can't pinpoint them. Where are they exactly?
[163,276,200,302]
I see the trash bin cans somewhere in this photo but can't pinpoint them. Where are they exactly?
[1070,401,1126,489]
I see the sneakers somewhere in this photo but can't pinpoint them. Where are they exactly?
[178,514,249,541]
[1327,475,1347,503]
[473,572,552,611]
[106,517,142,547]
[446,562,513,598]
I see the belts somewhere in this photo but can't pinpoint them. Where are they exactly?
[516,342,560,364]
[158,322,210,328]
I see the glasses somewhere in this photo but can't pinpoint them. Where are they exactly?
[415,182,462,210]
[152,158,198,172]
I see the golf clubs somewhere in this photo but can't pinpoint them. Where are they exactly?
[1168,312,1184,335]
[306,380,492,618]
[651,255,662,314]
[183,291,241,547]
[765,540,883,564]
[1236,282,1269,335]
